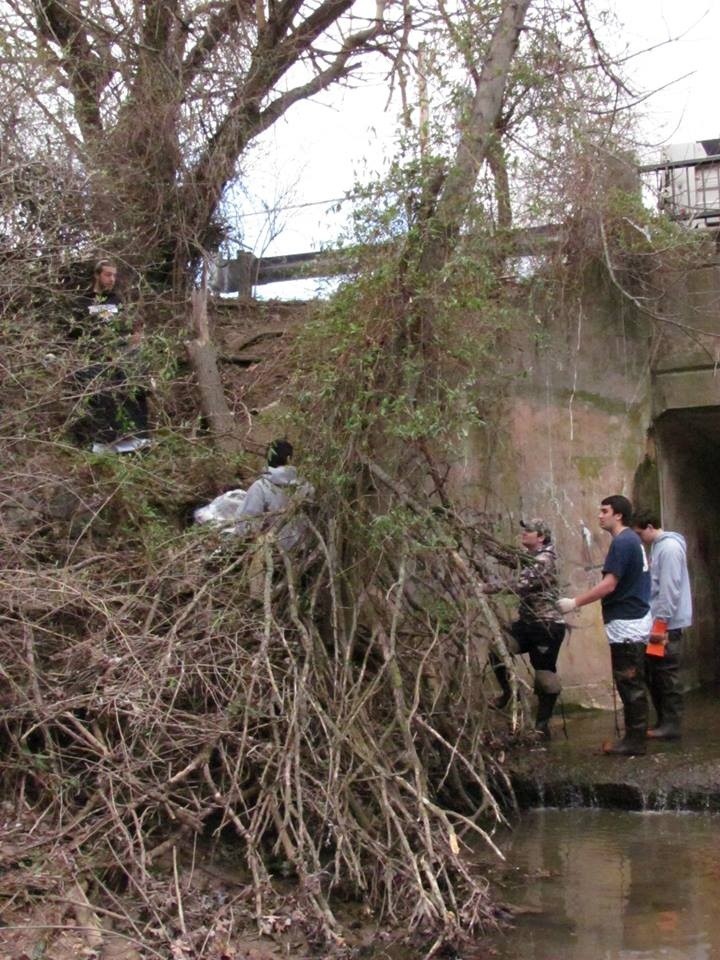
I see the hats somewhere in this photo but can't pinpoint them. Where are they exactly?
[520,519,550,539]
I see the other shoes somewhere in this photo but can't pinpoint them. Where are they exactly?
[119,438,152,452]
[488,690,520,709]
[92,443,117,457]
[527,724,552,742]
[602,736,648,753]
[646,724,679,738]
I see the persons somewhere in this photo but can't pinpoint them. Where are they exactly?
[467,517,566,743]
[555,496,692,756]
[234,438,315,608]
[64,257,152,454]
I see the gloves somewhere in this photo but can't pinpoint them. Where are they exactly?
[556,597,576,613]
